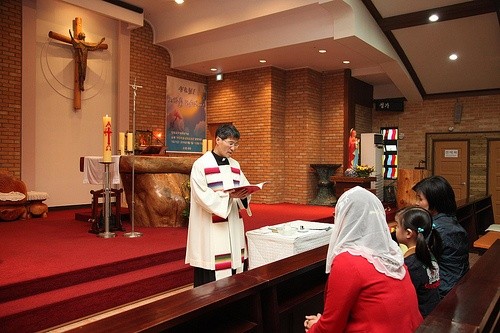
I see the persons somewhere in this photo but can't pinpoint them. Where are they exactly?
[303,185,425,333]
[348,130,360,170]
[69,29,106,91]
[394,206,441,320]
[184,124,252,289]
[412,176,471,300]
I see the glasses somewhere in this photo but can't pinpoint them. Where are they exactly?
[223,139,239,149]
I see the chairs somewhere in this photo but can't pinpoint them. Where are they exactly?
[90,188,126,235]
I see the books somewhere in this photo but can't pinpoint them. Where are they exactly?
[223,182,270,196]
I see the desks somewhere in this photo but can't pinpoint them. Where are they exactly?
[310,164,342,205]
[245,220,334,271]
[28,199,48,219]
[414,238,500,333]
[80,156,201,228]
[330,176,377,199]
[64,194,492,333]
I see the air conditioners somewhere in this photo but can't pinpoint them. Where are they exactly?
[361,133,384,202]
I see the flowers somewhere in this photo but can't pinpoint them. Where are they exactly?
[345,164,374,177]
[180,182,191,218]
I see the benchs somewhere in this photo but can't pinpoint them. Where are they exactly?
[474,231,500,255]
[0,170,27,221]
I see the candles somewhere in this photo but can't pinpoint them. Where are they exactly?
[208,140,212,151]
[202,139,207,152]
[119,132,125,150]
[103,114,112,162]
[127,133,133,151]
